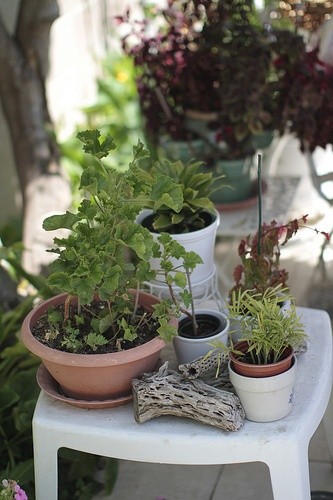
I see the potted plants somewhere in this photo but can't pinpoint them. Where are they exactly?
[201,285,309,422]
[113,0,333,233]
[21,130,327,409]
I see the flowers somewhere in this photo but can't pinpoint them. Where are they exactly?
[0,478,26,500]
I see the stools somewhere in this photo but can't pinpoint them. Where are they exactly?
[32,306,332,499]
